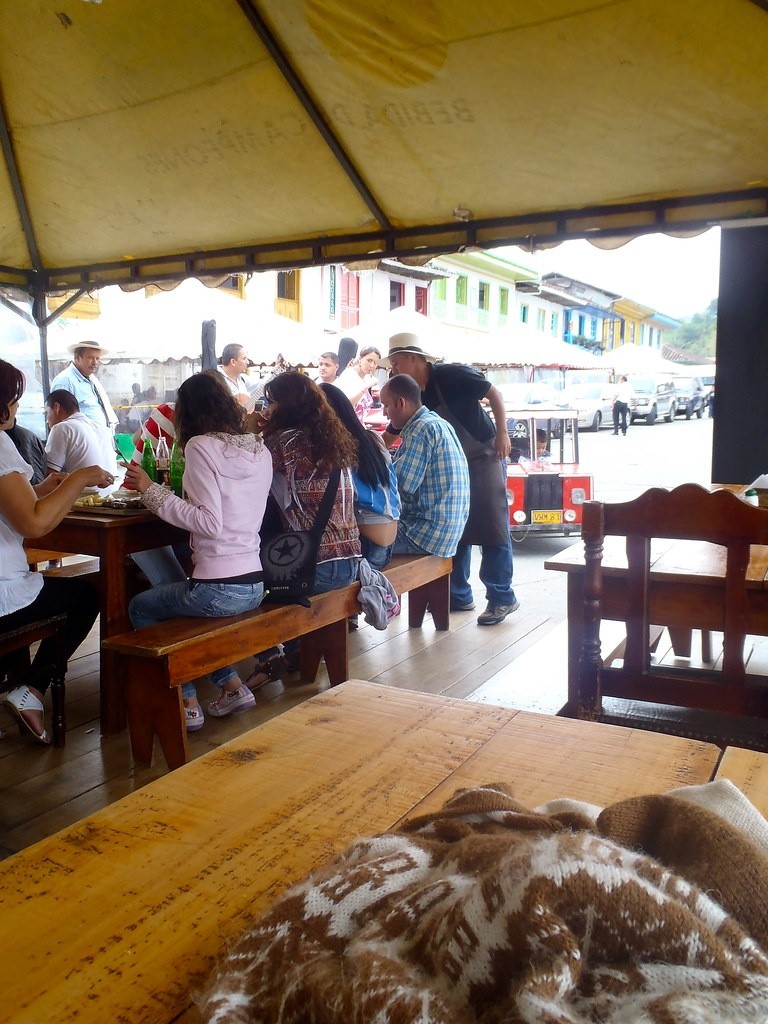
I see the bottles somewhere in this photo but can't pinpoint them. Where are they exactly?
[156,437,170,491]
[745,489,759,506]
[170,438,186,499]
[141,439,156,483]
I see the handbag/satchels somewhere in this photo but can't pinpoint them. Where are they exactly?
[259,530,320,608]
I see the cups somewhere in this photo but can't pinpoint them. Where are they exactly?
[371,386,381,403]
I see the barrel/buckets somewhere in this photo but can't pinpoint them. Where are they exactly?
[115,433,136,459]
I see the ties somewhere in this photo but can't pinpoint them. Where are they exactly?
[94,385,110,427]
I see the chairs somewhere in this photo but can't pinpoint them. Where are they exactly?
[577,483,768,754]
[0,611,68,747]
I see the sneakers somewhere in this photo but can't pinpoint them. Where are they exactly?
[208,685,256,717]
[425,601,475,612]
[181,704,205,732]
[477,594,520,626]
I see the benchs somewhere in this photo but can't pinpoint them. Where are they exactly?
[508,437,532,463]
[100,553,453,770]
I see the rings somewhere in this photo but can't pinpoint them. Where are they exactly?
[108,476,111,481]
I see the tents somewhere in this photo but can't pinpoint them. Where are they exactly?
[0,278,342,364]
[337,305,615,389]
[0,0,768,409]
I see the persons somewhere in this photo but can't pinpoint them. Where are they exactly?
[118,373,272,728]
[0,359,113,744]
[315,383,402,570]
[379,374,470,558]
[2,416,46,487]
[520,429,551,461]
[610,375,630,435]
[375,332,520,625]
[216,343,251,397]
[708,386,715,417]
[242,372,364,692]
[328,345,381,424]
[50,340,120,439]
[42,389,117,491]
[336,337,358,378]
[311,352,340,388]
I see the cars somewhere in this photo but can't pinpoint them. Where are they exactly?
[479,402,596,557]
[480,364,717,440]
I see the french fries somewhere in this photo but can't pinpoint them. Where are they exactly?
[74,494,114,507]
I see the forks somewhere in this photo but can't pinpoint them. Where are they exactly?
[112,436,129,464]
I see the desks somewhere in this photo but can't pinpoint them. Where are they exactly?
[1,678,768,1024]
[21,512,169,737]
[544,483,768,719]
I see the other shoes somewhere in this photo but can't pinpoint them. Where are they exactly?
[623,433,626,436]
[348,618,359,631]
[611,433,618,435]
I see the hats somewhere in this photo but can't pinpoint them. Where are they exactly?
[378,333,436,368]
[68,341,108,356]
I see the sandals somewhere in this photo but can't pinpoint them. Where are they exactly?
[4,686,53,745]
[279,648,300,672]
[243,655,289,690]
